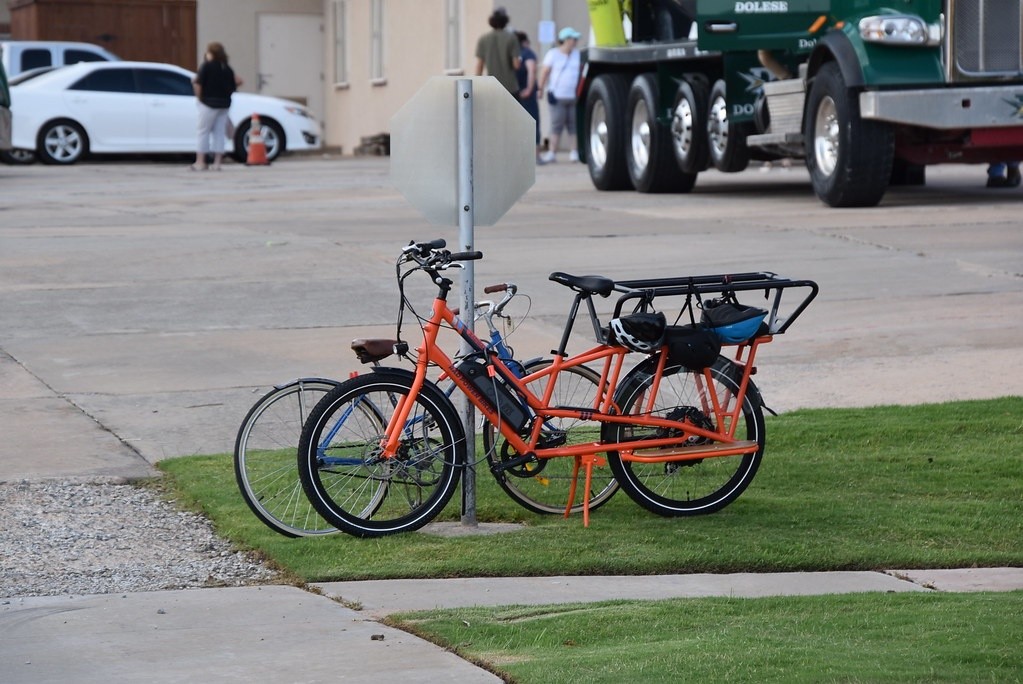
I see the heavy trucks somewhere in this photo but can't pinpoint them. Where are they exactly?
[570,0,1023,209]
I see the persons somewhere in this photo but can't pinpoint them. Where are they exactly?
[514,31,545,164]
[537,27,583,164]
[475,7,522,101]
[986,160,1021,189]
[189,42,236,172]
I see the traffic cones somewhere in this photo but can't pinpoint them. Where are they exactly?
[242,112,272,166]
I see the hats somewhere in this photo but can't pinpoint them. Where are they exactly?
[559,27,581,41]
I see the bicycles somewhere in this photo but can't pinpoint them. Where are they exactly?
[230,281,636,539]
[297,238,822,539]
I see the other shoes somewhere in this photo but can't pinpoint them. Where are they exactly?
[570,152,579,161]
[543,152,555,162]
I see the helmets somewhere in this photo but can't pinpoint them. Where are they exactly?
[609,312,667,354]
[668,325,722,369]
[700,299,768,345]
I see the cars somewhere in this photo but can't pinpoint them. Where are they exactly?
[6,59,326,168]
[0,40,130,80]
